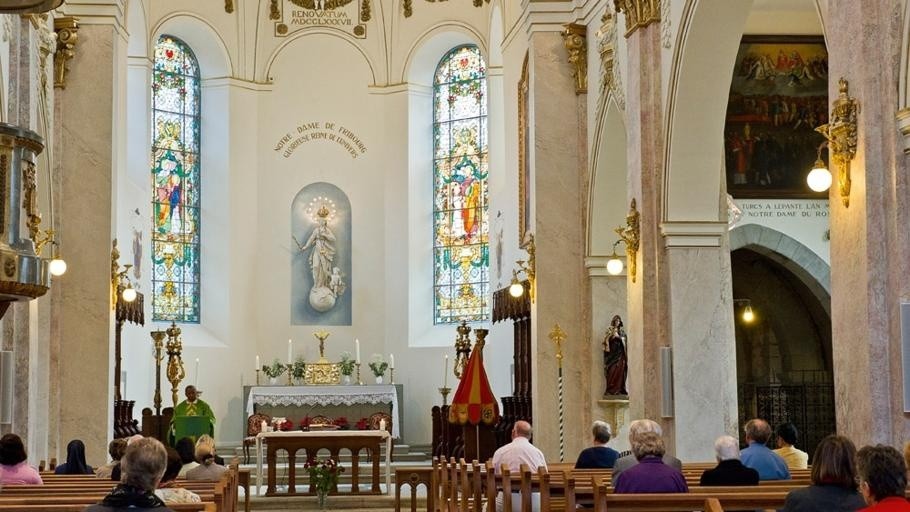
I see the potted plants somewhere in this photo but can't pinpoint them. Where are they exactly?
[261,356,288,387]
[336,350,356,385]
[363,352,388,384]
[291,350,307,386]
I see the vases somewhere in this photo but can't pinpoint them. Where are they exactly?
[315,488,329,510]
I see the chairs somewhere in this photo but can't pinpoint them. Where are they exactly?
[307,414,333,431]
[363,411,395,464]
[241,412,271,467]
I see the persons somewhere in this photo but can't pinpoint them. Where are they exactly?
[700,435,759,486]
[572,418,621,508]
[600,314,628,394]
[0,432,43,485]
[328,267,341,297]
[151,444,202,504]
[614,432,689,493]
[735,419,789,480]
[122,431,229,483]
[852,442,910,511]
[769,423,810,469]
[169,384,216,439]
[481,419,548,511]
[55,439,93,473]
[777,434,869,511]
[77,437,174,512]
[612,418,681,481]
[299,218,333,286]
[95,437,127,478]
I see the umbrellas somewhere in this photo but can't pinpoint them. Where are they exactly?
[447,344,499,464]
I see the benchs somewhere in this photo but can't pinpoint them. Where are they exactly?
[0,461,253,512]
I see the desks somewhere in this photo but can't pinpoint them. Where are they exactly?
[252,427,395,499]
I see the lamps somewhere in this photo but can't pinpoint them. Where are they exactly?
[110,235,137,311]
[25,204,68,278]
[731,293,755,327]
[607,195,642,283]
[509,231,536,305]
[805,75,862,212]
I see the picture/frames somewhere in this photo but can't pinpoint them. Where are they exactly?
[719,31,831,204]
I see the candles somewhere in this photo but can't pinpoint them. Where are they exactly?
[287,339,293,362]
[354,338,361,364]
[442,353,449,388]
[389,352,394,369]
[255,355,259,370]
[192,357,200,391]
[379,416,385,431]
[260,417,268,433]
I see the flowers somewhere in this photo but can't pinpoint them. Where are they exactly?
[300,453,347,511]
